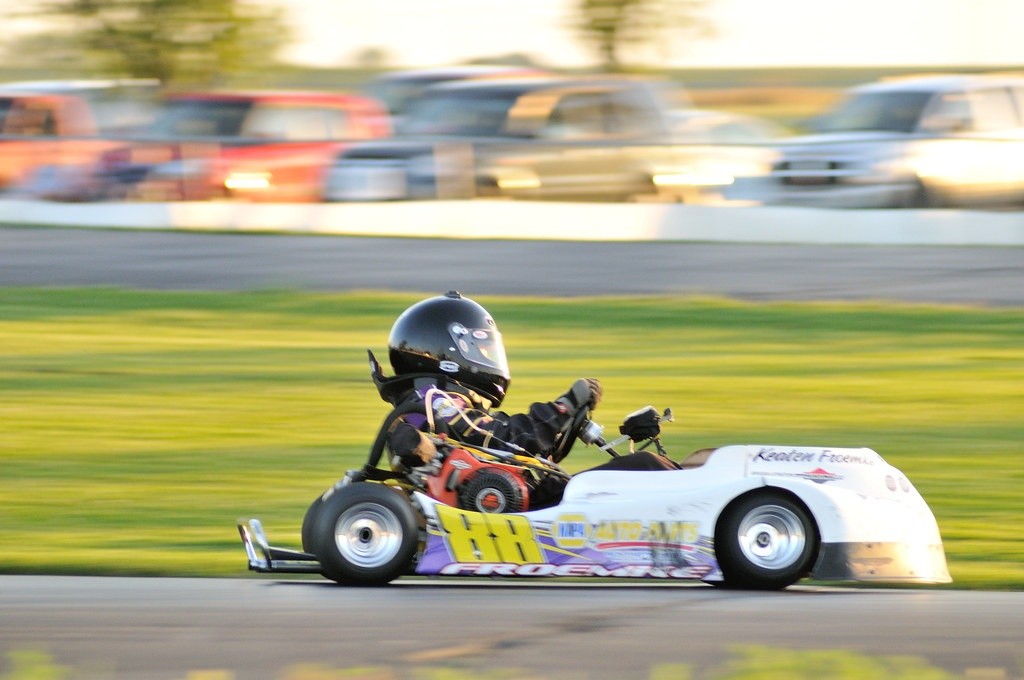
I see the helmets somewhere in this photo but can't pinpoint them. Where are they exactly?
[388,290,510,408]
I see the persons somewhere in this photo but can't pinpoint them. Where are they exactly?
[383,290,684,513]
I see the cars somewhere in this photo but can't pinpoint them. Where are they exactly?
[363,64,534,115]
[1,79,174,204]
[94,89,393,205]
[663,108,800,184]
[323,76,700,204]
[720,75,1024,209]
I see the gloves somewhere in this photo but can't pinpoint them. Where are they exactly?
[555,377,601,417]
